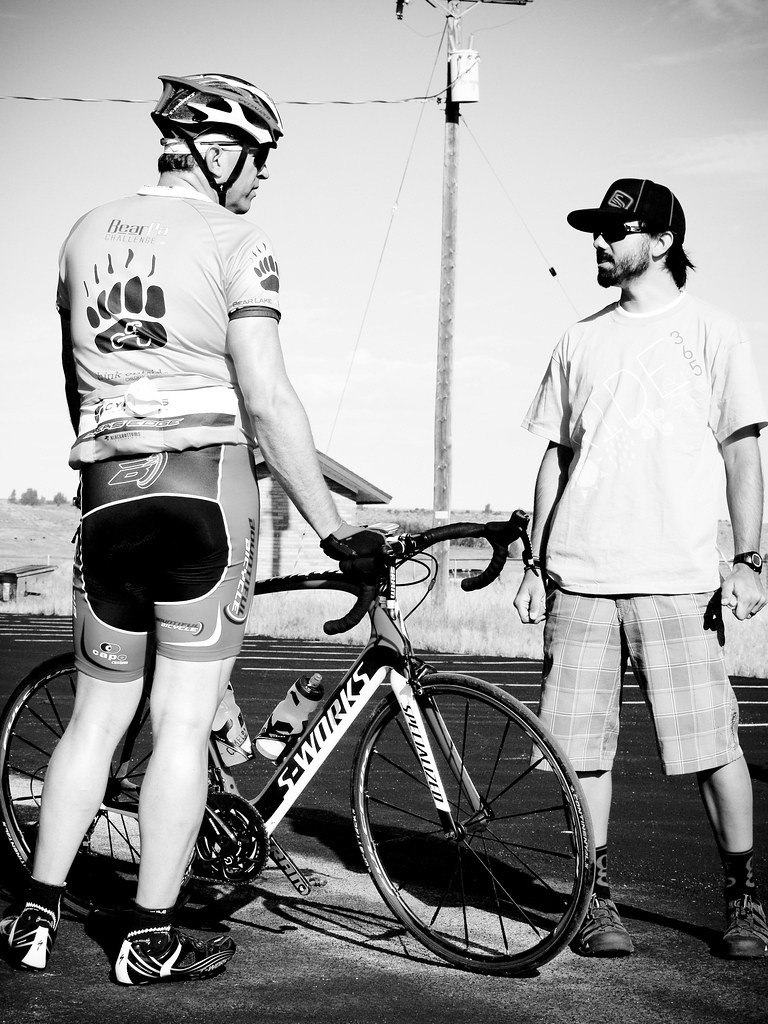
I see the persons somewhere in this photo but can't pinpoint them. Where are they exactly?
[515,177,768,958]
[1,73,396,986]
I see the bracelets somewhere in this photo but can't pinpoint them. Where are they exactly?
[524,556,543,573]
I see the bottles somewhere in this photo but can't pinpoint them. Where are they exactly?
[256,673,324,761]
[212,681,254,768]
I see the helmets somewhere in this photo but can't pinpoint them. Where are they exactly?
[151,72,284,149]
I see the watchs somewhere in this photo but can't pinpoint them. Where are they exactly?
[733,551,763,574]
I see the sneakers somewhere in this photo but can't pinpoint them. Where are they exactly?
[721,893,768,957]
[109,922,237,987]
[0,915,58,974]
[576,892,635,953]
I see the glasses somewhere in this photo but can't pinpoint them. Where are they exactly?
[200,141,269,171]
[591,220,648,245]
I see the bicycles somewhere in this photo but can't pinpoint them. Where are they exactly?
[0,509,598,978]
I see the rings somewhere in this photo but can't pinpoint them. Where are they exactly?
[750,612,755,617]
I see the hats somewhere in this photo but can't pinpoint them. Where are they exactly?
[566,178,686,242]
